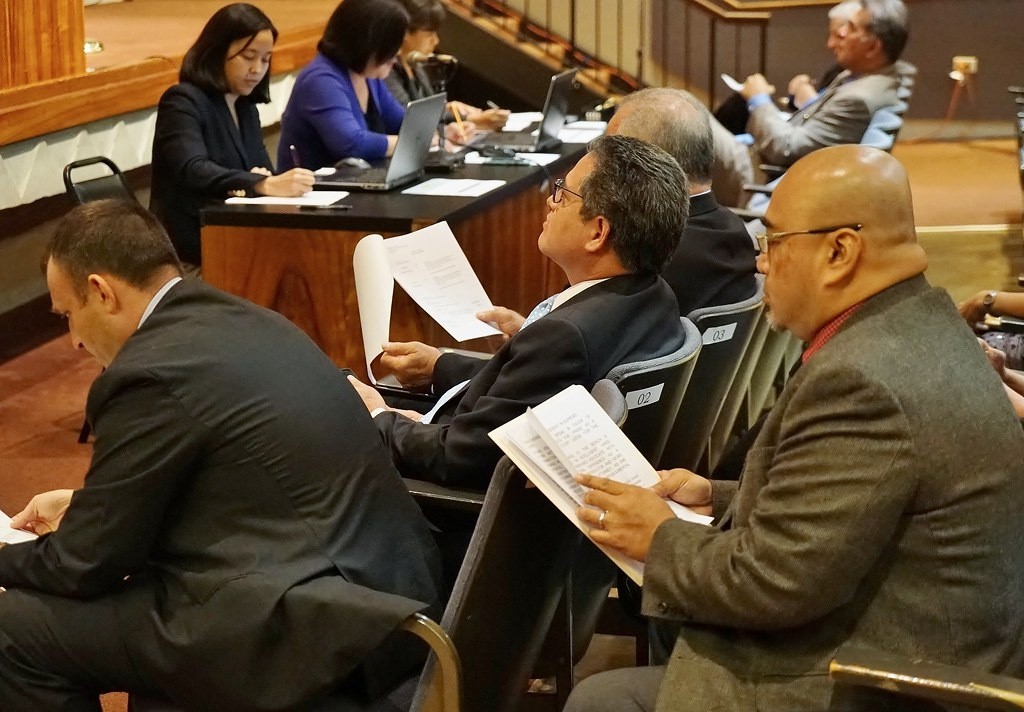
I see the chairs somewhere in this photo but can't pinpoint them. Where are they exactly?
[63,57,1024,712]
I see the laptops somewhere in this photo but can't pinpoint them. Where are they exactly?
[471,68,578,153]
[313,92,447,190]
[375,347,492,400]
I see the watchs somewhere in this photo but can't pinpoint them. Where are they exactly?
[983,290,1001,318]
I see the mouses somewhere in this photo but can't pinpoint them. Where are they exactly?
[334,157,371,169]
[478,143,516,158]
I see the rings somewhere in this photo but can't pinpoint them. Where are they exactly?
[599,510,608,529]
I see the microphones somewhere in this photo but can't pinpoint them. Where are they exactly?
[406,51,456,66]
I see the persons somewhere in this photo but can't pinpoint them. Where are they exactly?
[477,88,757,341]
[0,198,444,712]
[149,3,316,267]
[347,134,686,605]
[955,290,1023,420]
[714,1,909,167]
[383,0,511,130]
[277,0,476,176]
[564,144,1024,712]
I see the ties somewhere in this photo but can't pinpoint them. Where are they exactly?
[519,293,561,332]
[712,357,802,480]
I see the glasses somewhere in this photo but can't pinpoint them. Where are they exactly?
[756,223,862,254]
[586,141,592,151]
[553,178,584,203]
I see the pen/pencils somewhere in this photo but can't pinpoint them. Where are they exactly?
[487,101,499,109]
[290,144,300,167]
[295,205,353,209]
[451,103,466,140]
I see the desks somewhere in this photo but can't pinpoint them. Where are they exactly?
[199,112,608,395]
